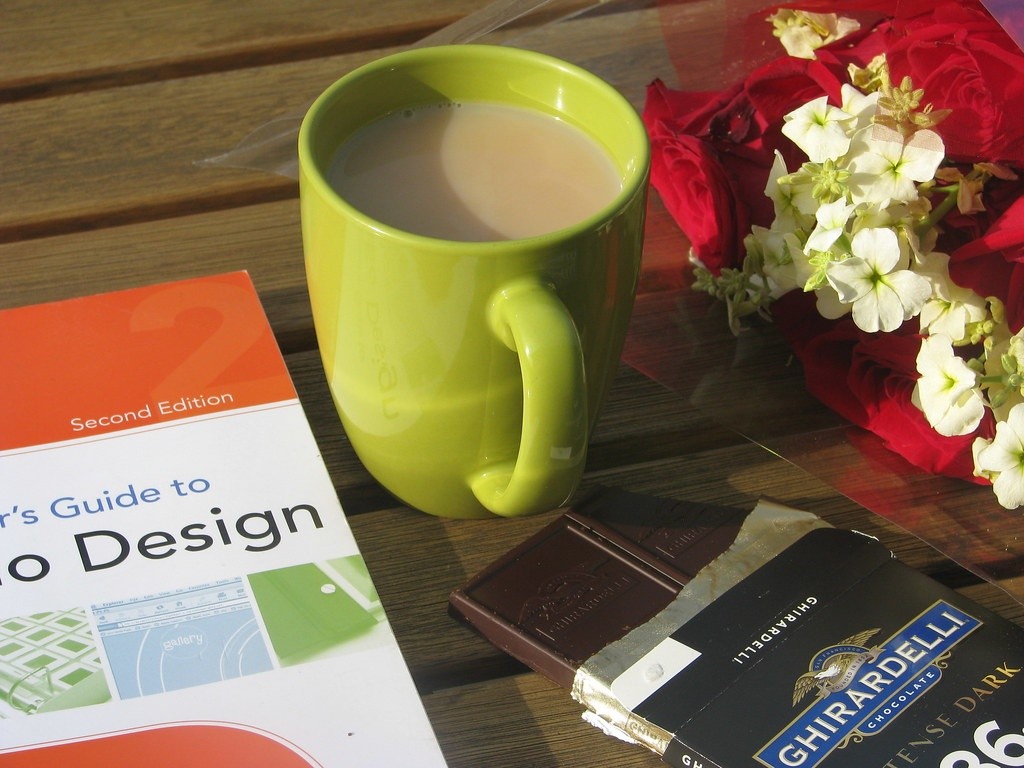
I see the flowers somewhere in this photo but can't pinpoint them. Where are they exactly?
[687,6,1024,511]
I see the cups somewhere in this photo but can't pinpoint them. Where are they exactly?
[299,44,652,519]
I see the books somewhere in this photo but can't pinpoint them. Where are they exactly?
[0,269,450,768]
